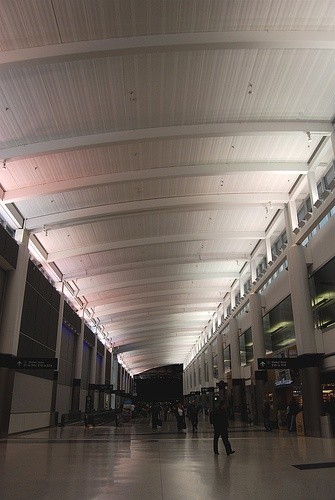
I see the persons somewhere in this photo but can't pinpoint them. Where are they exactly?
[213,400,235,456]
[143,402,198,433]
[287,397,298,432]
[175,403,186,433]
[264,402,273,432]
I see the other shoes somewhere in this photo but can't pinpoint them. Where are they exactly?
[215,452,220,455]
[227,450,236,455]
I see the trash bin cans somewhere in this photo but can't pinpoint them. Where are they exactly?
[287,414,295,432]
[320,414,334,439]
